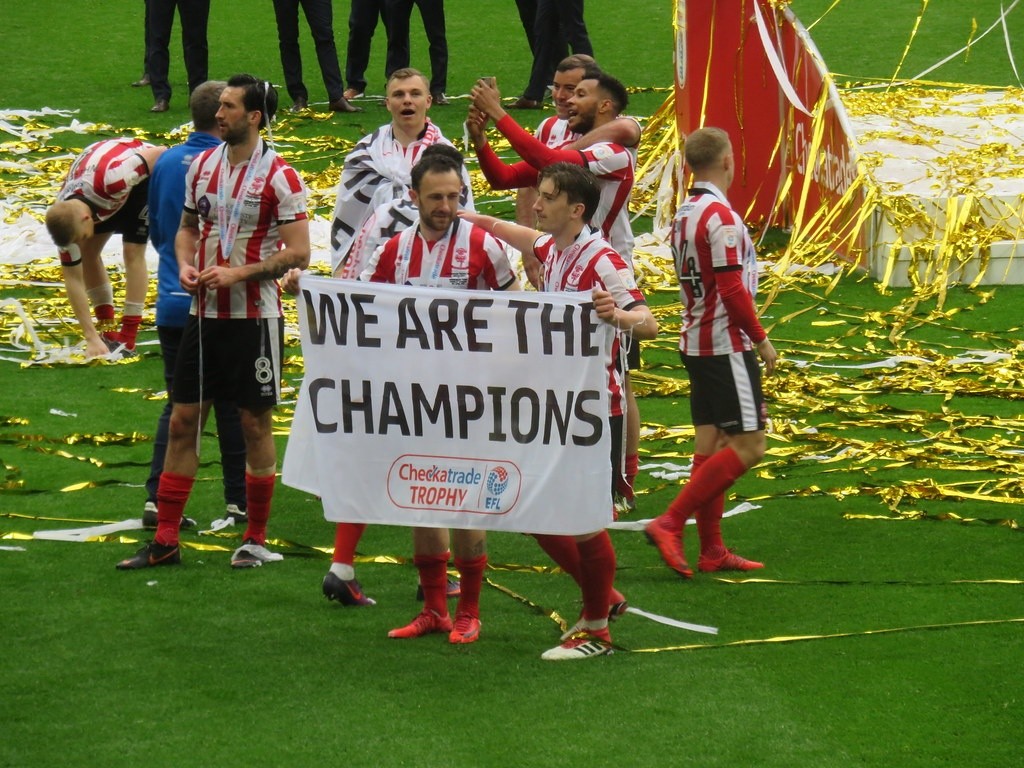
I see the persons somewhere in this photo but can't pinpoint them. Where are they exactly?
[116,74,311,570]
[466,74,640,511]
[281,143,460,607]
[344,0,450,105]
[131,0,210,112]
[516,54,641,291]
[357,155,523,643]
[644,127,778,579]
[141,81,248,532]
[271,0,362,113]
[330,69,475,282]
[45,138,167,357]
[504,0,594,109]
[458,160,658,661]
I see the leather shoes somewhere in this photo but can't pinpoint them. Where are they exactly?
[328,97,364,112]
[503,95,544,109]
[131,76,151,86]
[291,97,308,113]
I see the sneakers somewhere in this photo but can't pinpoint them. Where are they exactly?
[414,579,461,600]
[141,500,198,530]
[560,594,629,642]
[386,606,458,638]
[696,546,764,573]
[98,335,118,354]
[225,502,252,526]
[230,537,284,565]
[447,610,482,644]
[539,633,614,662]
[109,341,140,360]
[644,518,695,581]
[116,542,182,570]
[323,569,375,606]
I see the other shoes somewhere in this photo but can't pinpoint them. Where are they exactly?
[342,86,365,102]
[149,99,169,113]
[429,90,452,104]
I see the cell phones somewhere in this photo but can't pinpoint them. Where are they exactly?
[481,77,492,87]
